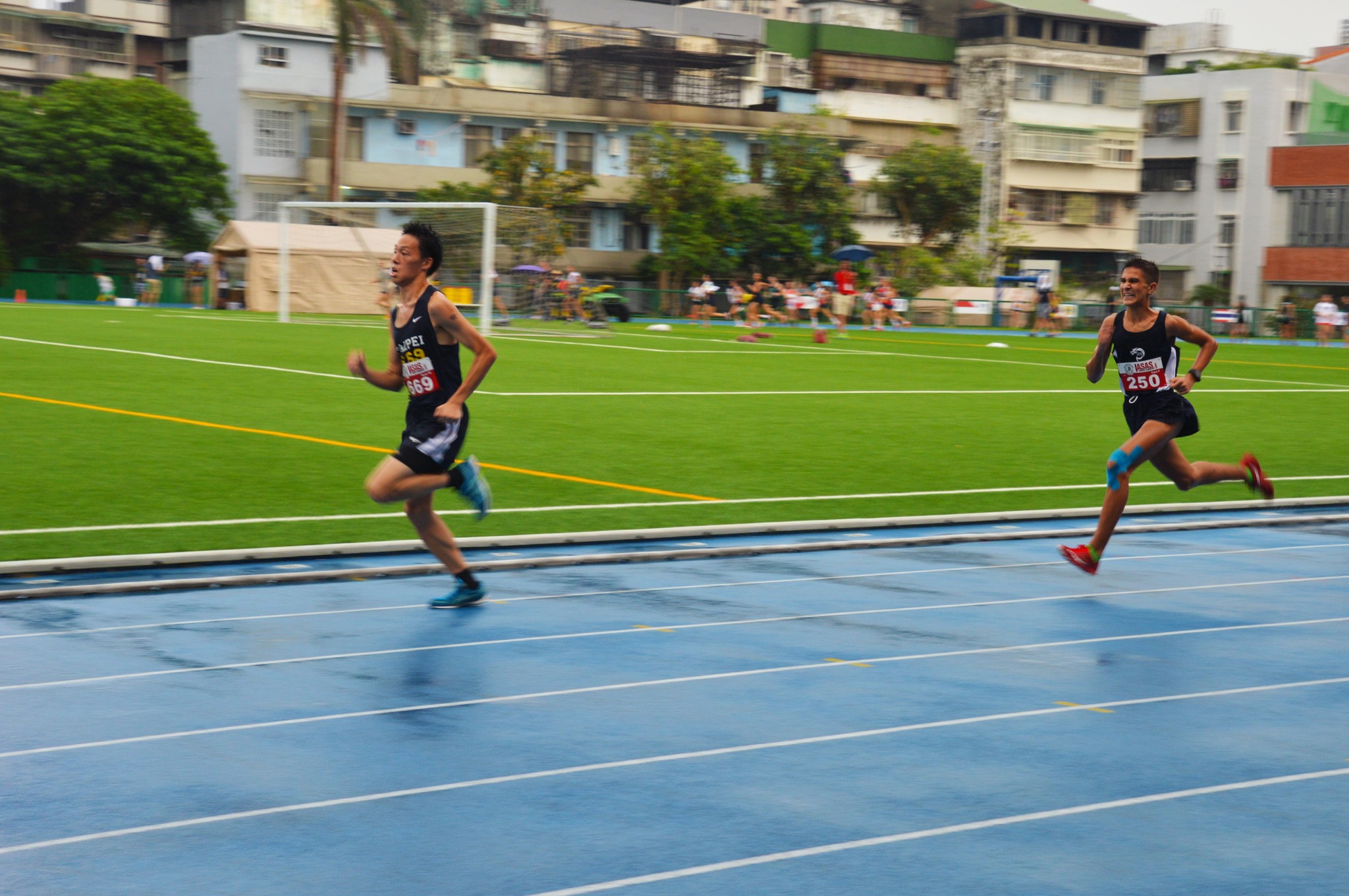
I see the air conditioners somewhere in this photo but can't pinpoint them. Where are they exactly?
[1174,180,1192,191]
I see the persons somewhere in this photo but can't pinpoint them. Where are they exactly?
[685,257,913,342]
[136,250,234,312]
[1029,268,1058,336]
[1058,258,1275,576]
[347,221,498,609]
[1229,293,1349,345]
[1101,290,1118,318]
[503,262,596,324]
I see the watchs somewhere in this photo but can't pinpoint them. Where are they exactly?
[1187,368,1204,385]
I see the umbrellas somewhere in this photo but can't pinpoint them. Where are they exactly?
[826,244,874,272]
[508,264,550,276]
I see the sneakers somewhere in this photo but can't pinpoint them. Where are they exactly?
[451,454,493,521]
[1054,542,1100,575]
[1238,452,1277,501]
[425,579,490,609]
